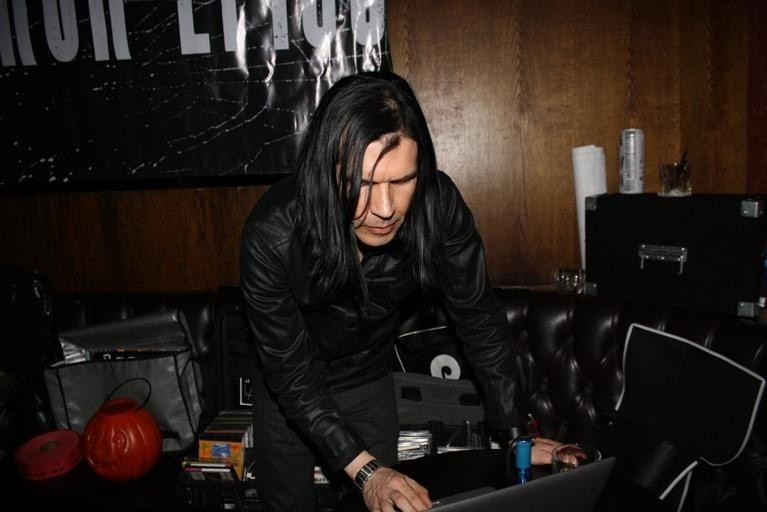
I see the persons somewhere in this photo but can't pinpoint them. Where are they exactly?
[238,70,591,511]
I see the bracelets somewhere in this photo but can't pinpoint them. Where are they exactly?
[352,456,388,494]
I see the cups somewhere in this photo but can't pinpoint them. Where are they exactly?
[551,267,586,293]
[551,443,602,473]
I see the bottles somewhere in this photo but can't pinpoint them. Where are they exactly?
[513,433,536,489]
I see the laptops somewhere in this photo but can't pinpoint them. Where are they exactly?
[398,457,617,512]
